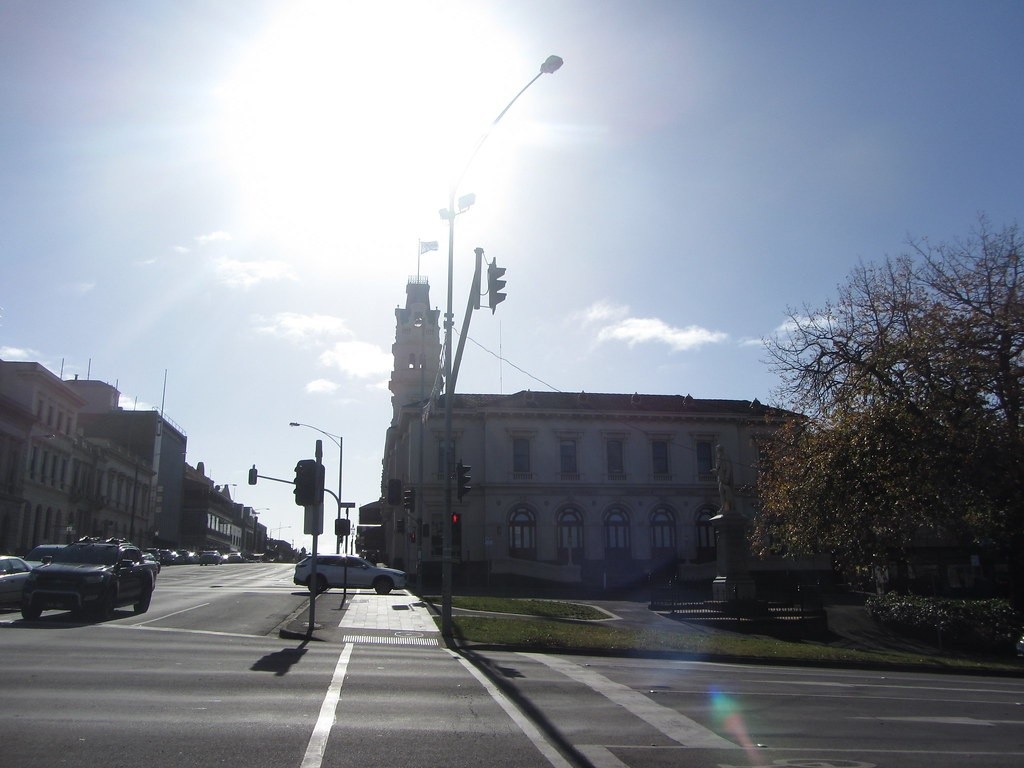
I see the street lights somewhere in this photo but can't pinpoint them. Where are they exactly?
[288,421,342,554]
[437,54,565,635]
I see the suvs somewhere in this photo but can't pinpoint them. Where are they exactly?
[293,554,408,594]
[21,538,155,620]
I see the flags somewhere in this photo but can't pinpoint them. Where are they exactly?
[420,241,438,254]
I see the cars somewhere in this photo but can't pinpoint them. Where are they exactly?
[200,550,224,566]
[138,552,161,578]
[146,547,199,565]
[23,544,69,575]
[223,552,246,563]
[0,556,34,609]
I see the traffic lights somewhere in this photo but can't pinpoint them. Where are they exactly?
[404,488,416,513]
[293,459,315,506]
[476,248,508,314]
[248,469,258,486]
[451,513,462,547]
[456,458,472,501]
[398,518,406,535]
